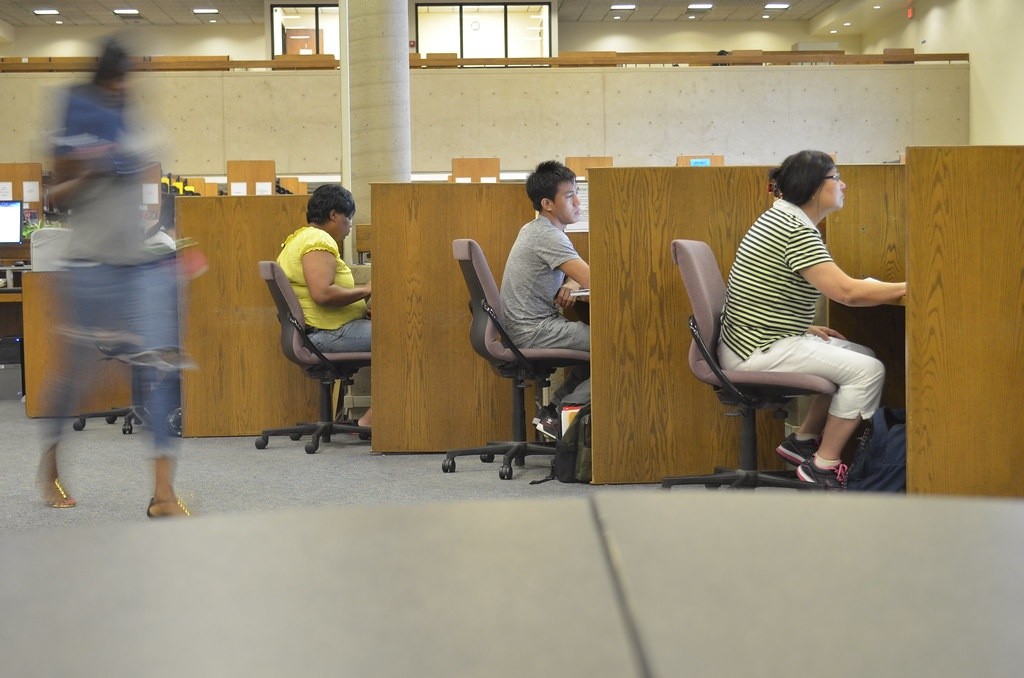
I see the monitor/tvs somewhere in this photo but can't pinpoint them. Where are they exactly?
[0,200,23,245]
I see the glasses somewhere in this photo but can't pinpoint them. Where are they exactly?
[825,172,841,183]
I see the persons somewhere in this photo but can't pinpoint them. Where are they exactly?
[716,147,907,485]
[499,160,591,440]
[33,33,190,518]
[277,185,372,428]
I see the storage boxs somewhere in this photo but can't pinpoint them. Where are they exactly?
[343,395,372,421]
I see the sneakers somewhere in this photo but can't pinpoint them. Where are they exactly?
[796,457,847,490]
[532,403,555,425]
[537,415,559,440]
[775,432,822,465]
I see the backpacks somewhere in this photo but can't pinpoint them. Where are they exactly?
[553,402,592,483]
[848,407,906,492]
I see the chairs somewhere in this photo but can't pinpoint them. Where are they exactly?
[72,363,146,434]
[441,237,591,480]
[256,260,372,454]
[662,239,838,491]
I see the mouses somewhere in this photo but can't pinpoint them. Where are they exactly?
[15,260,24,267]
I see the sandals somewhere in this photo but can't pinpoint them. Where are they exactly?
[146,497,192,520]
[35,472,75,508]
